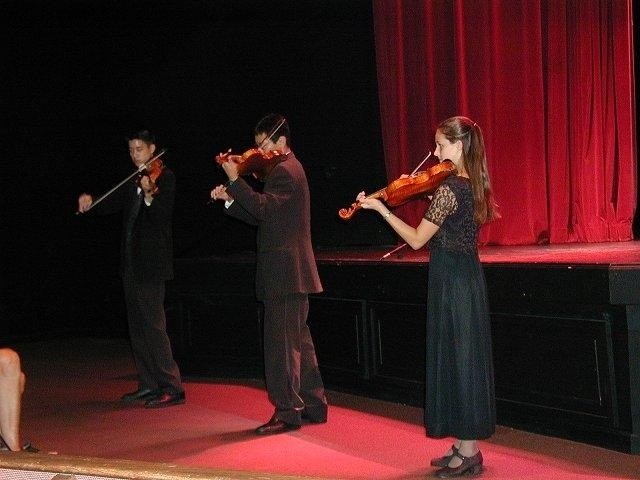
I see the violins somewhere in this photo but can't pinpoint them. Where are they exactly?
[216,148,284,183]
[138,157,163,196]
[339,160,458,219]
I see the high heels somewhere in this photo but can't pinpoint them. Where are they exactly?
[431,441,483,478]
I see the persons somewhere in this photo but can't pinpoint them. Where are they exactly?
[356,115,496,479]
[1,347,26,453]
[209,110,328,436]
[75,128,185,409]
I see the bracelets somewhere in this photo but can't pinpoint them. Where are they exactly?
[383,210,392,220]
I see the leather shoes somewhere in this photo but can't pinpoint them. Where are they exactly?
[143,392,185,407]
[121,389,159,404]
[257,400,328,434]
